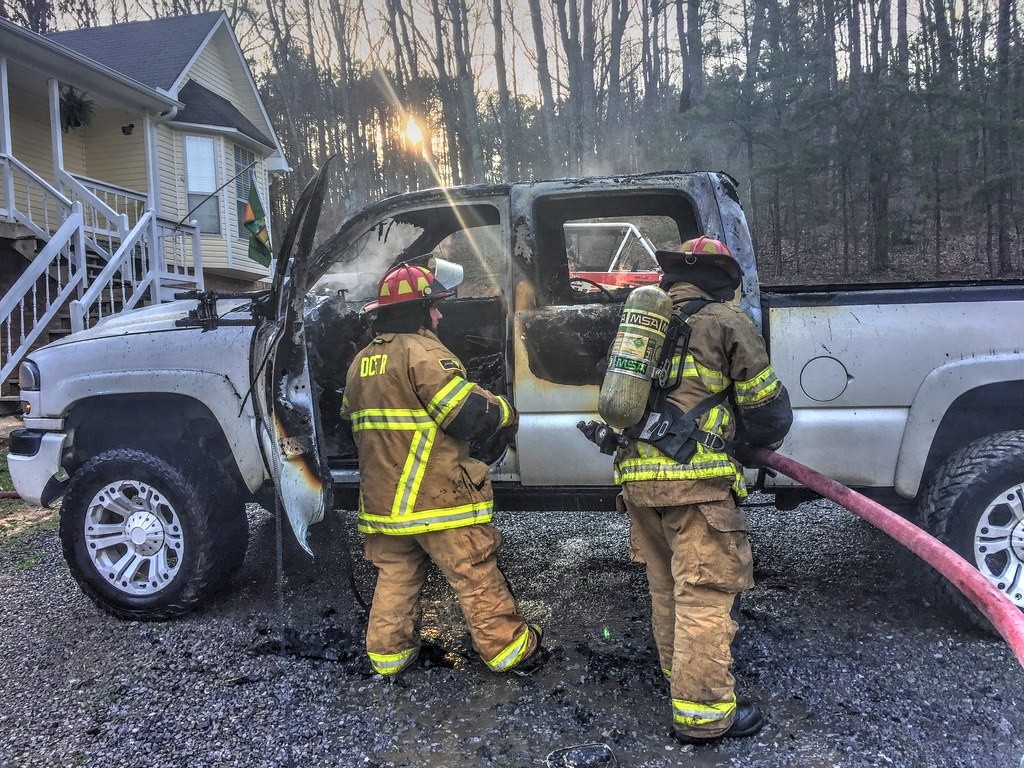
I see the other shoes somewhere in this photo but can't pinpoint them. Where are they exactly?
[384,642,475,688]
[670,703,764,745]
[508,644,588,678]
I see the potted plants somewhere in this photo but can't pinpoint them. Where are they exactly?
[60,86,96,134]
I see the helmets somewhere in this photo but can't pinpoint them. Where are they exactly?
[654,236,743,289]
[359,261,456,317]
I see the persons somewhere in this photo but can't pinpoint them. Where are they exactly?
[596,235,793,746]
[339,263,545,676]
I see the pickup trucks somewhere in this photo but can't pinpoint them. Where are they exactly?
[5,153,1024,626]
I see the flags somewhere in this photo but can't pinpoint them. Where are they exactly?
[246,167,272,267]
